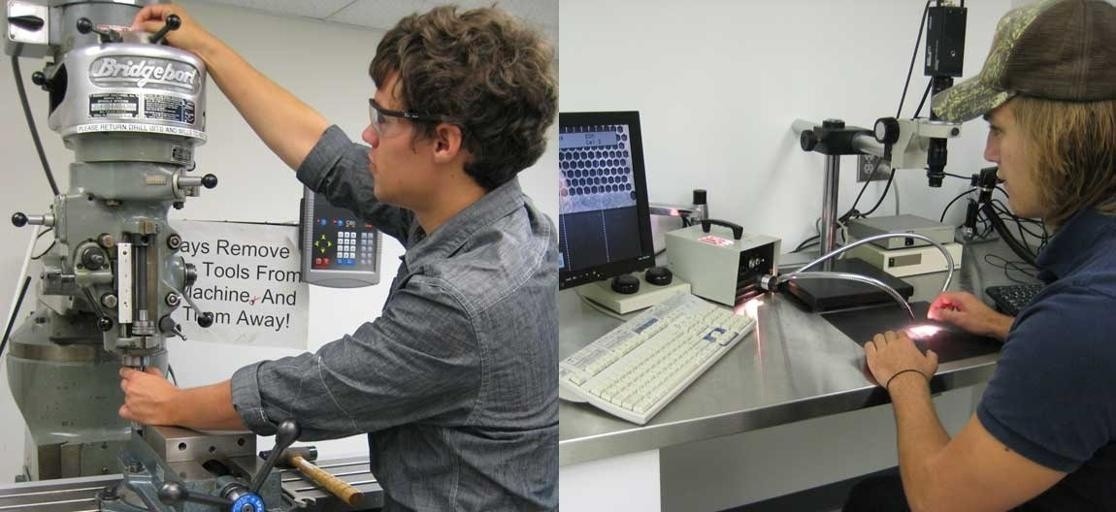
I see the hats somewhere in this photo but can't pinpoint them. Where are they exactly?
[931,0,1116,122]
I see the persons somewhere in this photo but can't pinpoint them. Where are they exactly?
[117,3,560,512]
[865,1,1116,509]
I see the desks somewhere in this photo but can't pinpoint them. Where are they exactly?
[558,216,1048,511]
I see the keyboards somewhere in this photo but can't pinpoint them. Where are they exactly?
[984,281,1053,317]
[559,292,757,426]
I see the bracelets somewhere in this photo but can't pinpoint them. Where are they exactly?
[888,368,929,391]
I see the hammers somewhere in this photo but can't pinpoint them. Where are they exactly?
[259,446,365,508]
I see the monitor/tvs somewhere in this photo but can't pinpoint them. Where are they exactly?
[560,111,657,289]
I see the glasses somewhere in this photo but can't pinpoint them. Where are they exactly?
[369,99,454,135]
